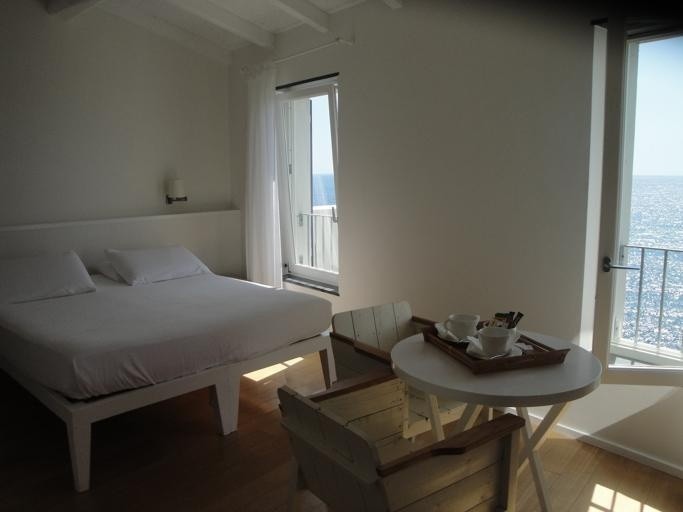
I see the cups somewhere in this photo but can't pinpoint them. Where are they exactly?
[470,326,509,357]
[483,319,515,340]
[444,312,480,339]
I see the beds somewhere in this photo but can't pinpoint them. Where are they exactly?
[0,240,332,494]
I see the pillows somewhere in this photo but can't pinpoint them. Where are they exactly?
[104,243,212,286]
[0,251,97,305]
[86,260,125,282]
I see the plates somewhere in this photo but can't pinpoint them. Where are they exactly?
[467,343,525,359]
[433,321,478,345]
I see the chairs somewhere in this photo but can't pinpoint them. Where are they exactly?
[275,373,527,512]
[328,301,475,439]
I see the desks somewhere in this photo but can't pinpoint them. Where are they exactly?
[388,329,604,511]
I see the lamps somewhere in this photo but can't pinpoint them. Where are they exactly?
[165,178,189,206]
